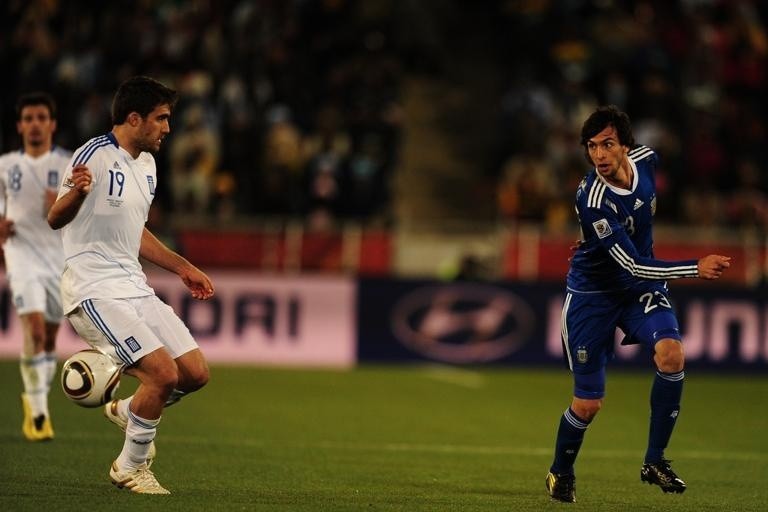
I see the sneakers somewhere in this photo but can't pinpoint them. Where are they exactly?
[640,460,687,495]
[546,472,576,504]
[104,398,132,433]
[108,459,171,495]
[21,393,54,443]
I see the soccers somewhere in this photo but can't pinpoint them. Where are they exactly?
[61,352,120,408]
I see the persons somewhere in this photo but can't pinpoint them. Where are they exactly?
[546,104,730,502]
[46,77,216,493]
[0,90,76,442]
